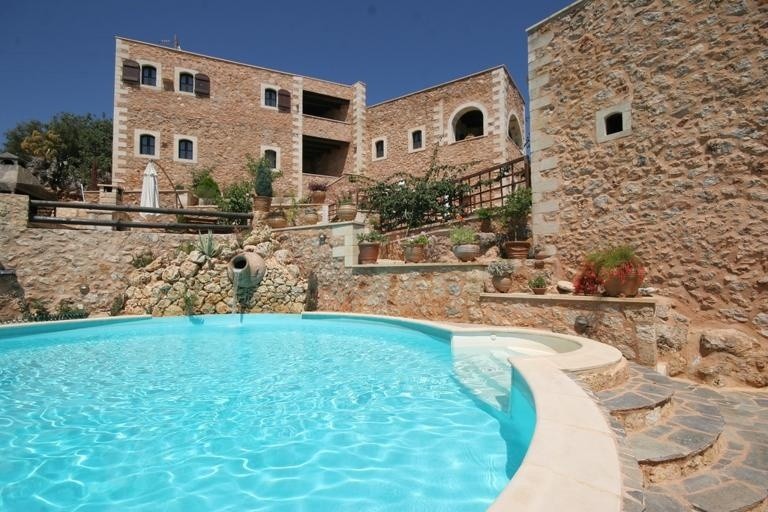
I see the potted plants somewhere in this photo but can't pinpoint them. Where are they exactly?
[492,184,533,259]
[399,234,428,263]
[354,230,388,265]
[570,242,647,297]
[449,223,481,262]
[526,276,548,295]
[487,259,514,293]
[190,151,357,229]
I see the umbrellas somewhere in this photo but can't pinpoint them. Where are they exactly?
[138,161,161,220]
